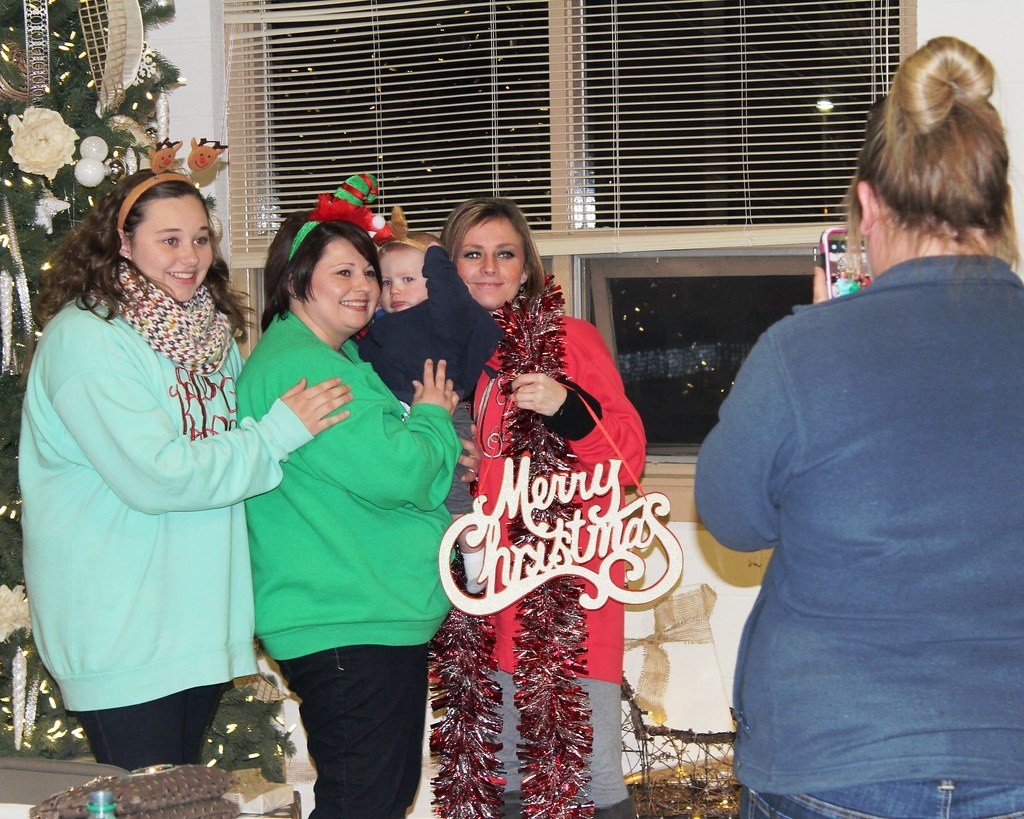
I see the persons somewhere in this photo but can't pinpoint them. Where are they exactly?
[695,36,1024,819]
[836,269,870,298]
[236,209,483,819]
[355,232,502,594]
[433,198,646,819]
[18,169,354,770]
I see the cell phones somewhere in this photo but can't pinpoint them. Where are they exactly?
[820,227,873,300]
